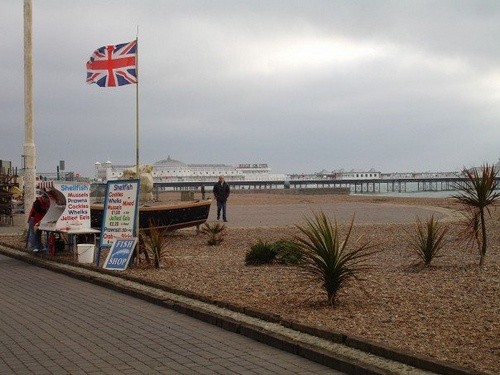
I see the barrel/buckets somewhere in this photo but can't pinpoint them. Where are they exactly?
[77,244,95,264]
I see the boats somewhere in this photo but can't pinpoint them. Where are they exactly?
[91,198,211,235]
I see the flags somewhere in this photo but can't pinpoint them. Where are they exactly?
[86,39,139,88]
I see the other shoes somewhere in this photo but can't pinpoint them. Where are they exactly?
[33,248,39,251]
[223,217,227,221]
[217,215,220,220]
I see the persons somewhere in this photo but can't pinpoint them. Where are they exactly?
[212,175,230,222]
[27,192,51,253]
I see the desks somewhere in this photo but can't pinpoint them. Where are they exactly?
[37,228,101,263]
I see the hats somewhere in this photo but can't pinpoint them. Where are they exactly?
[42,192,51,198]
